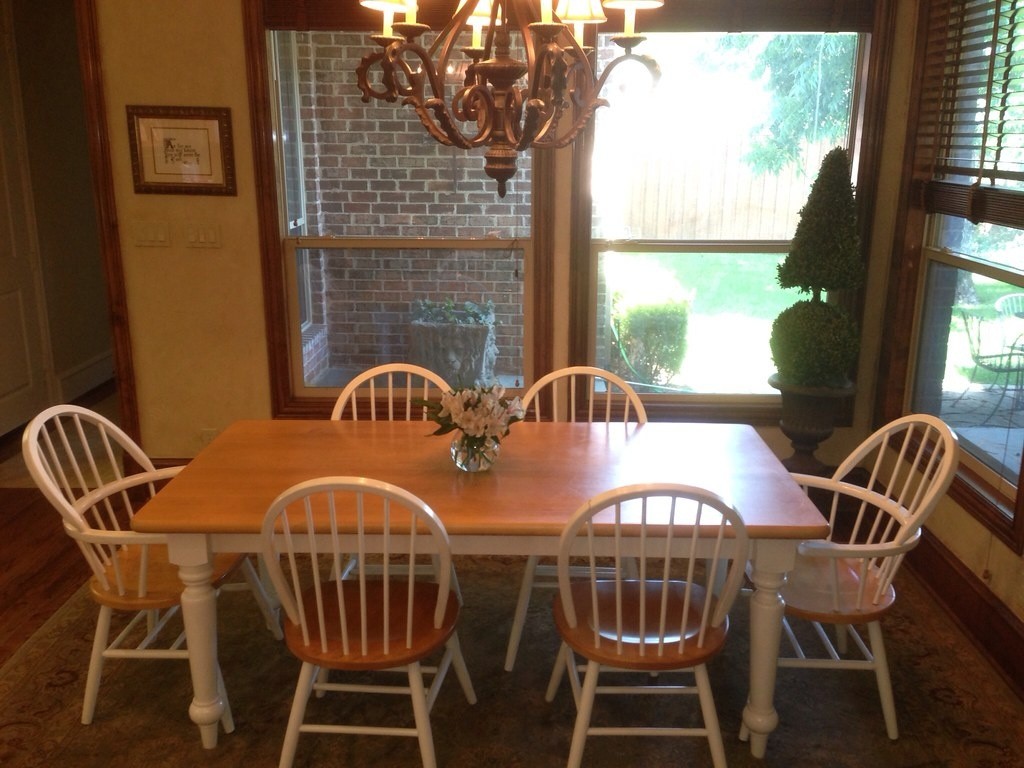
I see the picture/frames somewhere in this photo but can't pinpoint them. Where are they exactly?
[125,104,239,198]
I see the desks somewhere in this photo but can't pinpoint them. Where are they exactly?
[129,421,833,761]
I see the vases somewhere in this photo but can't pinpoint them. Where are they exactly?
[409,296,498,389]
[450,428,502,473]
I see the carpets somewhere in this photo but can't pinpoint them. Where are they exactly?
[0,531,1024,768]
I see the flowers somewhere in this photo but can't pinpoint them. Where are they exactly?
[408,366,526,468]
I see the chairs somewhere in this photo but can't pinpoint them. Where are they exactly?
[21,404,287,735]
[261,475,477,768]
[720,413,960,743]
[545,482,752,768]
[950,292,1024,427]
[330,361,467,612]
[503,364,659,677]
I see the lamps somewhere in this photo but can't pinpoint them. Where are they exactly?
[356,0,663,199]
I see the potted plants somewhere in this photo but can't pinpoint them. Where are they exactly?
[768,145,868,480]
